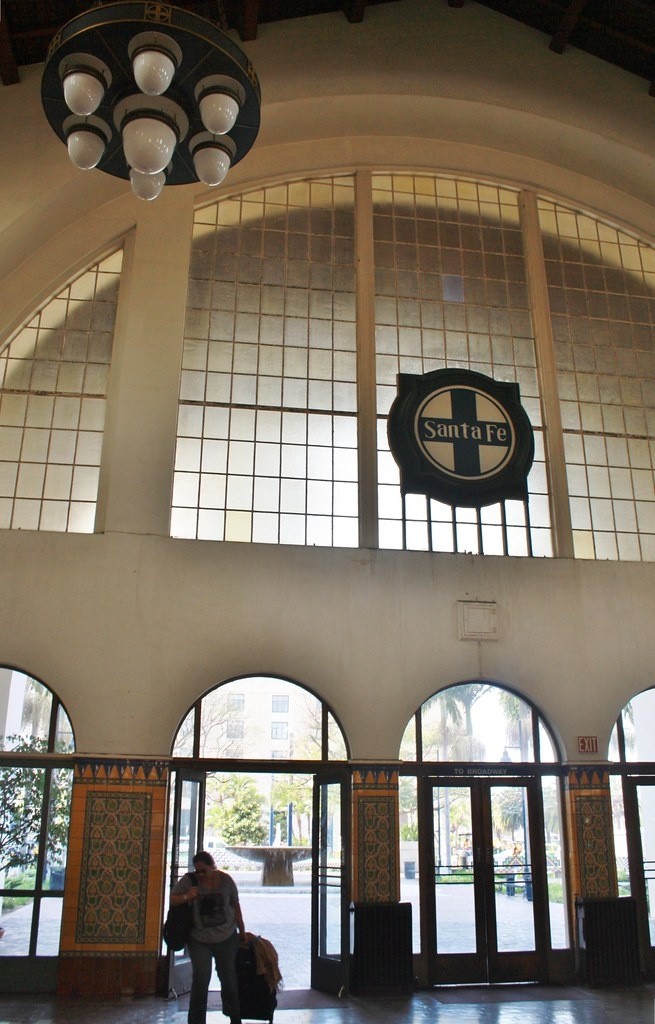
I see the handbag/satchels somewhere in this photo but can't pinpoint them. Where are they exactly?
[163,873,198,951]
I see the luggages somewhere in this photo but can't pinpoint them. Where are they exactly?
[235,933,281,1023]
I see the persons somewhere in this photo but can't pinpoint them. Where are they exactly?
[170,851,248,1024]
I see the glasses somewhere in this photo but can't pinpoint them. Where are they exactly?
[196,869,206,873]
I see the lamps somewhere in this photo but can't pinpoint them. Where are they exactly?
[40,0,263,201]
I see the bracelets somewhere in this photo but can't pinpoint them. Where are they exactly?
[184,893,188,901]
